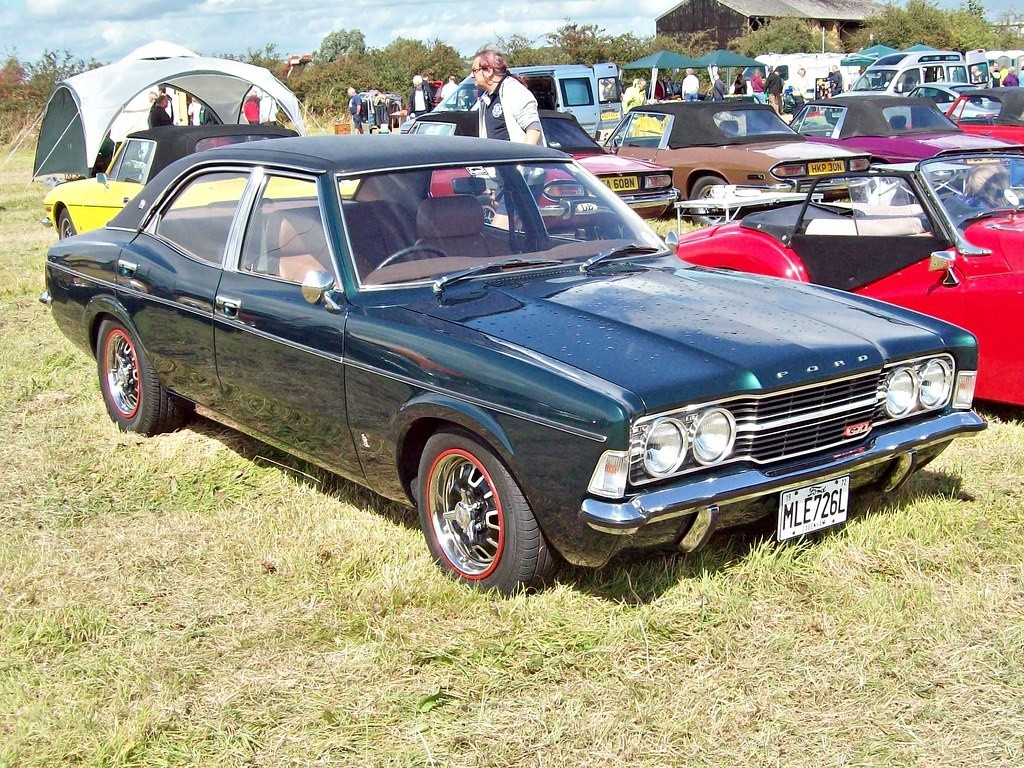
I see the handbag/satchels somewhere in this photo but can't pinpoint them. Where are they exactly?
[261,121,273,126]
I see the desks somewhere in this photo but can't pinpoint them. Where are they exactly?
[725,94,754,103]
[673,192,826,236]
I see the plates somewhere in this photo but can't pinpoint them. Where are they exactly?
[736,190,761,196]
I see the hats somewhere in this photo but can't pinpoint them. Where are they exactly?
[413,75,423,84]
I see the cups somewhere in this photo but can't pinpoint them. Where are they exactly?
[710,185,724,198]
[724,184,736,198]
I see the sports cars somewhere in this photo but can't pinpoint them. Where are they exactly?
[661,144,1023,411]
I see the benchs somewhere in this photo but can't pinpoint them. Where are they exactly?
[800,218,927,236]
[158,199,417,279]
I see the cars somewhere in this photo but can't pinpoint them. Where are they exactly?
[350,54,1024,235]
[41,132,991,596]
[41,127,359,255]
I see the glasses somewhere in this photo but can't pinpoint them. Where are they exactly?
[470,67,482,75]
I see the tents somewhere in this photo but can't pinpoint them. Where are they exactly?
[619,50,715,104]
[696,50,769,95]
[840,44,959,67]
[32,56,306,184]
[110,40,204,157]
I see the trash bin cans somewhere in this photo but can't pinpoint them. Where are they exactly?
[335,124,350,135]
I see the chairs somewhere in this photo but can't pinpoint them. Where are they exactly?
[889,115,909,130]
[257,206,333,283]
[904,77,915,86]
[719,120,739,136]
[414,193,513,261]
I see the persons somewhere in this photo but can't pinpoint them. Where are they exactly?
[348,87,364,135]
[916,68,928,85]
[99,136,115,172]
[830,65,843,97]
[953,62,1024,88]
[734,73,748,95]
[793,67,807,117]
[407,75,468,122]
[751,68,786,116]
[373,88,386,129]
[955,161,1019,210]
[856,70,870,90]
[148,84,219,129]
[712,72,726,102]
[240,89,279,126]
[655,74,668,99]
[682,68,699,102]
[600,78,647,137]
[472,42,548,231]
